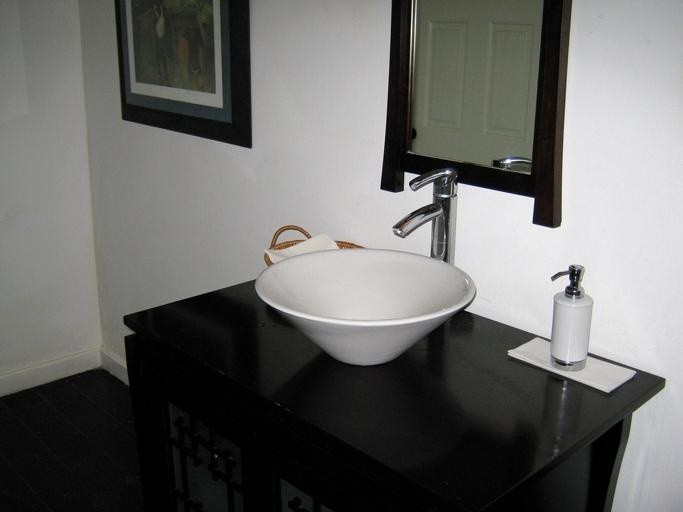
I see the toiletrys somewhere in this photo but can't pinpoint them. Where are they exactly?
[549,263,593,373]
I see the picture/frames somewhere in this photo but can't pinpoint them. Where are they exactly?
[112,0,253,147]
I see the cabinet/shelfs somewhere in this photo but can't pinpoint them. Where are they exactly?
[121,275,667,512]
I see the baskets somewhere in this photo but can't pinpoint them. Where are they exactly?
[264,225,360,266]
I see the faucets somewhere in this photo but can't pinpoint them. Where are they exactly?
[492,155,532,173]
[391,167,460,265]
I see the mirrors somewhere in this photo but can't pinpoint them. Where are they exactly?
[377,1,572,229]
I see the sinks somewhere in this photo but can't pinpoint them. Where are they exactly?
[253,246,477,368]
